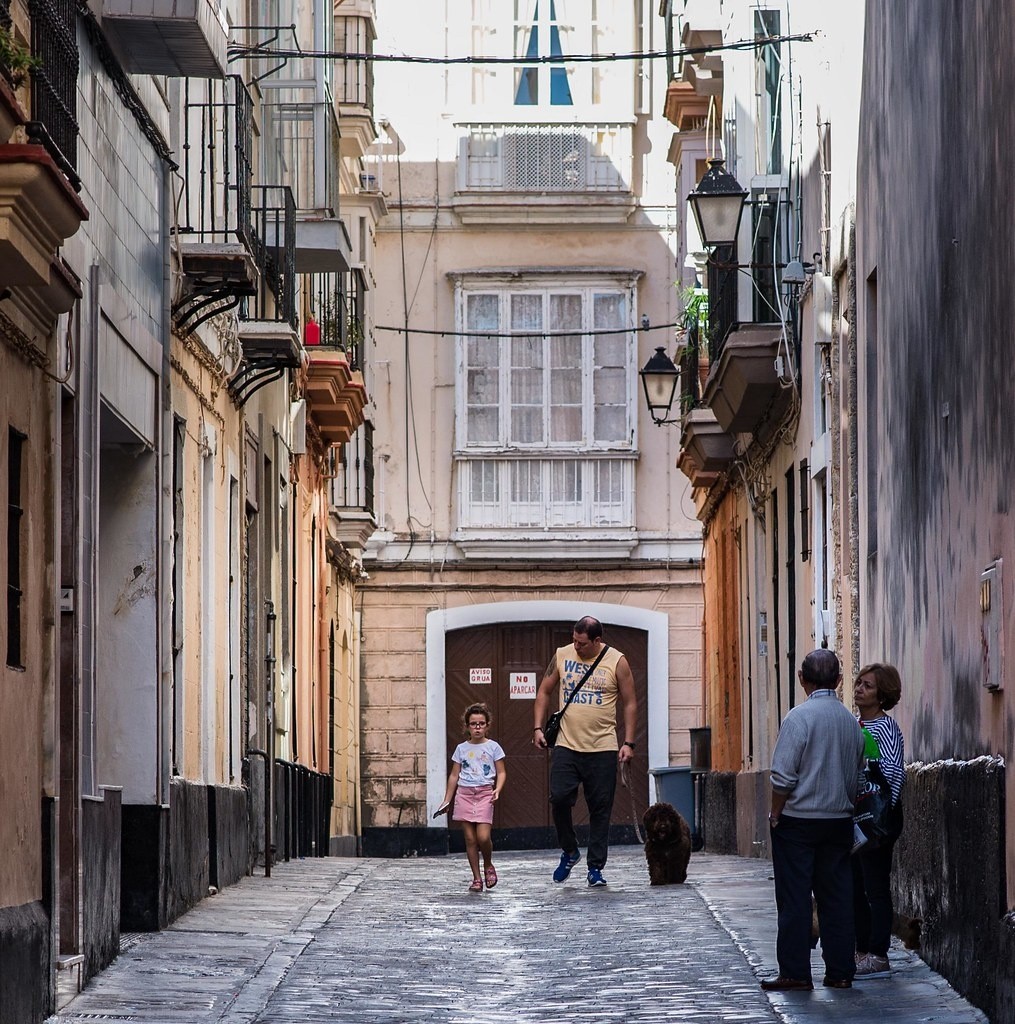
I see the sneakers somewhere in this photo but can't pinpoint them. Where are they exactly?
[586,868,607,887]
[552,848,582,884]
[853,951,892,980]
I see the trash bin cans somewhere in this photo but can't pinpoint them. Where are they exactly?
[648,765,701,849]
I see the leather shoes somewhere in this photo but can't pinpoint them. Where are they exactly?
[822,975,851,988]
[762,974,813,990]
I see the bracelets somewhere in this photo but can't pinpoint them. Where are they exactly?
[534,727,542,732]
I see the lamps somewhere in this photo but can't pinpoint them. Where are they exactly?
[782,261,817,283]
[638,348,682,429]
[687,157,815,267]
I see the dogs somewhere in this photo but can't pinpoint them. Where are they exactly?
[639,801,695,886]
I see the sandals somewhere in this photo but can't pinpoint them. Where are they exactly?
[485,867,497,888]
[469,881,483,892]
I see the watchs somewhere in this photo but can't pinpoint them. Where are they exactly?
[768,811,777,822]
[622,741,635,750]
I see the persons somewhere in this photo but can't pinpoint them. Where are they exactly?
[761,649,864,990]
[854,663,904,980]
[534,615,635,886]
[439,702,506,891]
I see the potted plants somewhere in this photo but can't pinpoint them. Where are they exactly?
[312,290,362,363]
[672,279,709,390]
[0,25,44,91]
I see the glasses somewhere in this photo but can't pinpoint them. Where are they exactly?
[468,722,487,728]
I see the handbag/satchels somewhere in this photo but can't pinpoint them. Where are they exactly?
[544,710,561,748]
